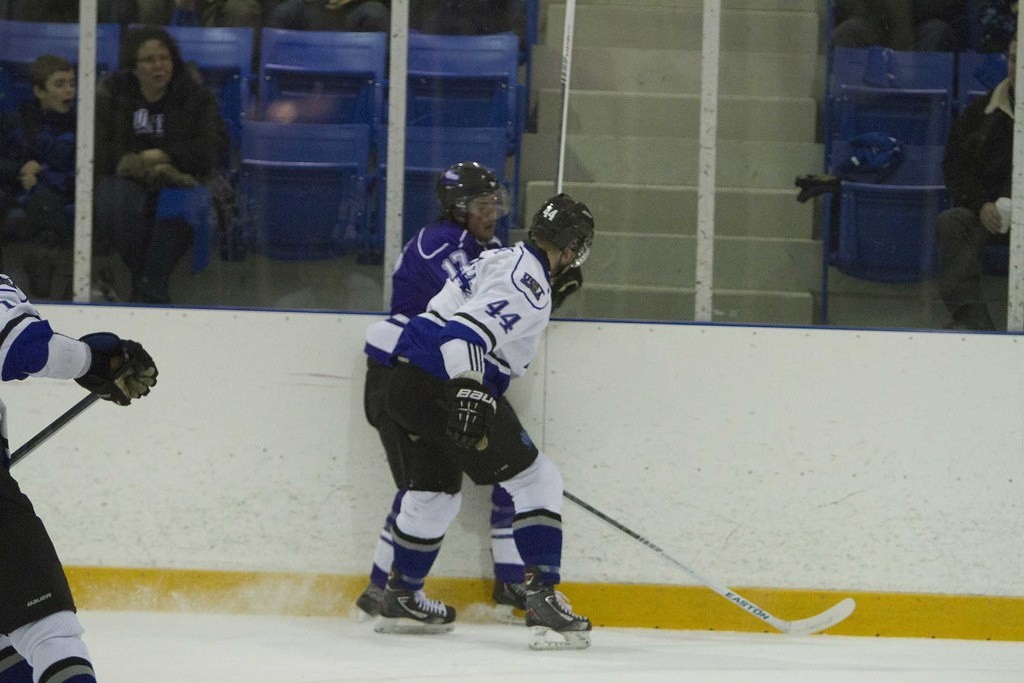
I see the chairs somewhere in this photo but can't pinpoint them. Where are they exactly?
[796,1,1019,327]
[0,0,542,267]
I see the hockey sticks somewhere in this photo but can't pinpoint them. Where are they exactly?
[562,489,858,637]
[8,342,144,470]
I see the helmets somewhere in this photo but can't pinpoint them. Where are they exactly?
[436,162,499,201]
[528,194,595,257]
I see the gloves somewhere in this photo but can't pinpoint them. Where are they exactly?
[550,266,582,310]
[447,384,498,451]
[75,333,159,406]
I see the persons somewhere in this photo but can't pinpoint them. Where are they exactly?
[0,0,525,304]
[352,163,528,629]
[0,271,158,683]
[829,0,1019,333]
[375,193,592,649]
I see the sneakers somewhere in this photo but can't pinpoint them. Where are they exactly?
[351,582,389,621]
[373,561,456,636]
[490,578,528,626]
[524,572,592,650]
